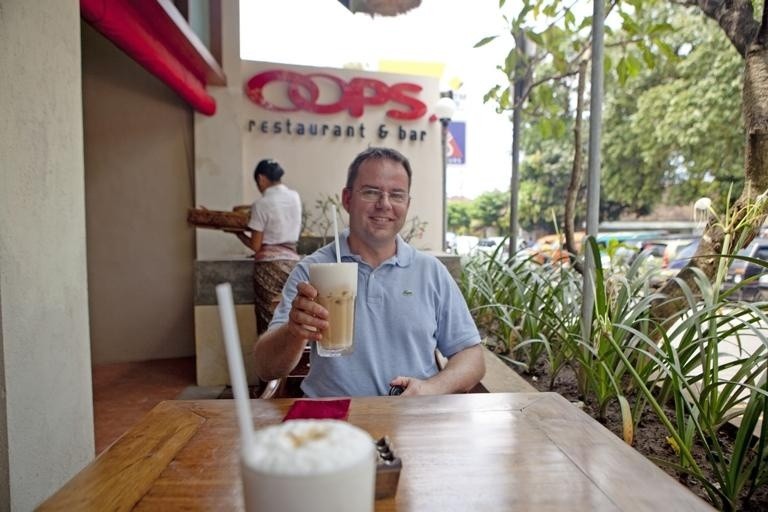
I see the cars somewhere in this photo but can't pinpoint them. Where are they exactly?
[523,231,768,312]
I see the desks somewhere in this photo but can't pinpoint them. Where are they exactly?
[28,391,719,511]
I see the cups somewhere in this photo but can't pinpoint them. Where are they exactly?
[308,262,358,358]
[241,419,379,511]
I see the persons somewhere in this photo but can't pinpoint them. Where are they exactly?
[222,158,302,391]
[253,147,488,397]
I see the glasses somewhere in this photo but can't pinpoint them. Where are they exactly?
[357,188,409,203]
